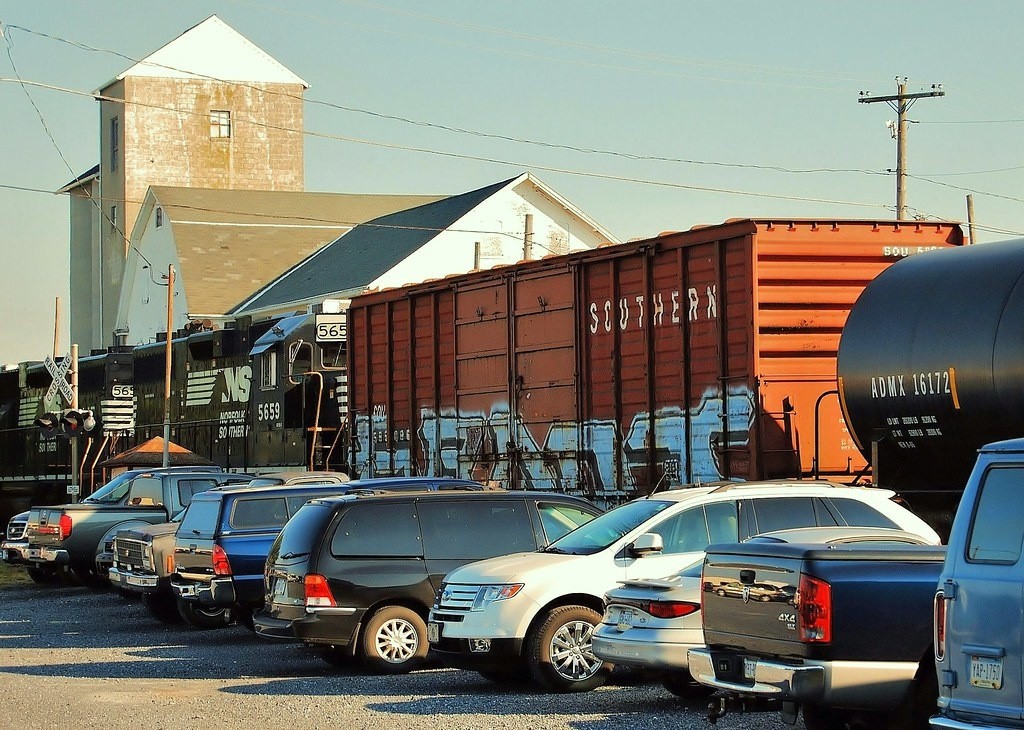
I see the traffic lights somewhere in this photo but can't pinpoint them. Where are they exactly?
[59,410,85,437]
[34,412,59,438]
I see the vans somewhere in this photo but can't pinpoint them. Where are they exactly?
[929,437,1024,730]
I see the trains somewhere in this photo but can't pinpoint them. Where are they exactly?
[0,219,1023,532]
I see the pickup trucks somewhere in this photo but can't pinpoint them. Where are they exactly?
[25,471,261,594]
[684,544,948,730]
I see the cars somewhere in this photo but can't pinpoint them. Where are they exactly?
[252,488,607,674]
[591,525,935,701]
[709,579,794,604]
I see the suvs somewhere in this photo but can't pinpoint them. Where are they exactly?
[107,472,355,627]
[170,476,497,630]
[0,465,223,587]
[426,480,943,695]
